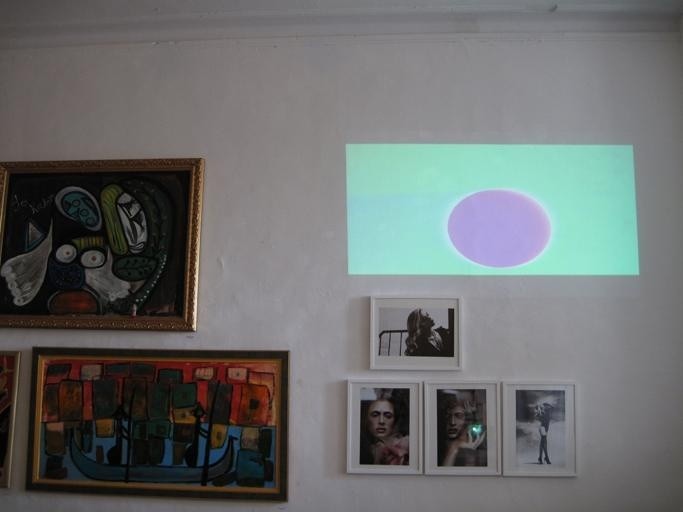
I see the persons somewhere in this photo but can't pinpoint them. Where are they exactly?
[438,390,486,467]
[532,397,558,464]
[359,394,408,466]
[403,308,449,356]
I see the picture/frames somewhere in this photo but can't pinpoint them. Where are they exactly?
[25,345,291,503]
[367,292,464,375]
[503,381,578,480]
[0,349,21,489]
[1,156,205,334]
[425,380,501,478]
[343,377,423,477]
